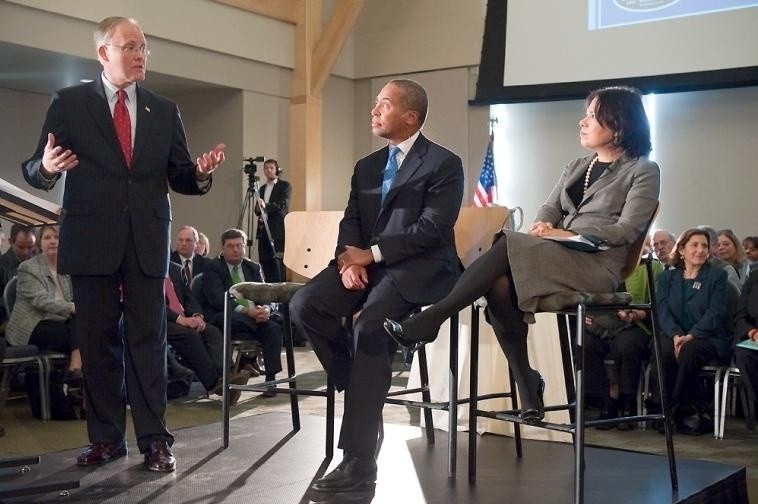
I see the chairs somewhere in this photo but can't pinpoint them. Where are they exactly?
[719,363,742,439]
[363,207,520,476]
[470,199,680,503]
[642,352,721,438]
[221,209,355,455]
[1,343,46,422]
[191,272,265,390]
[6,275,85,420]
[572,347,640,434]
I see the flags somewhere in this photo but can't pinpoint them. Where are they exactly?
[468,137,497,208]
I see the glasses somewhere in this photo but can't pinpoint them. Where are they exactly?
[104,43,151,56]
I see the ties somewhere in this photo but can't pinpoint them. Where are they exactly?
[164,276,184,314]
[231,265,249,308]
[113,90,132,167]
[184,260,191,285]
[380,146,400,208]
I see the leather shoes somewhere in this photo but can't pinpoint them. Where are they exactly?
[312,450,377,492]
[597,410,619,430]
[144,439,176,472]
[213,374,247,395]
[230,369,250,405]
[77,439,129,467]
[252,362,266,375]
[617,409,635,431]
[244,364,260,377]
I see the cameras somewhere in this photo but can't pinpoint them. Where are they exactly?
[244,154,266,174]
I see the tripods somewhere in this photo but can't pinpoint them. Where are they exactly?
[236,175,277,261]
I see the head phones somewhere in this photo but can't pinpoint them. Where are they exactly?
[268,161,284,176]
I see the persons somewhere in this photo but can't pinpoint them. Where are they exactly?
[195,231,209,257]
[651,231,677,269]
[290,80,464,493]
[656,229,736,437]
[21,16,226,472]
[167,225,209,285]
[583,247,664,431]
[384,88,660,423]
[204,229,282,397]
[0,220,38,321]
[6,225,83,397]
[734,268,758,435]
[741,235,758,262]
[716,229,750,283]
[696,224,742,297]
[164,261,248,405]
[254,159,292,282]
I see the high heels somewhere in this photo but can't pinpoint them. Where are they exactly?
[520,370,545,423]
[384,319,440,355]
